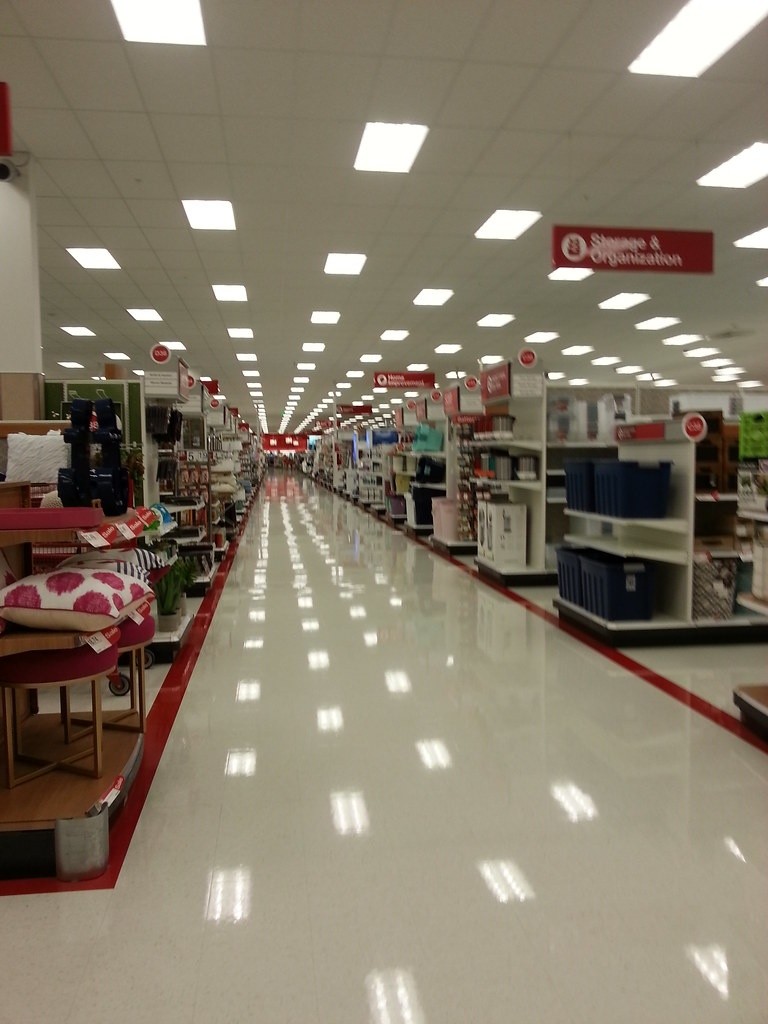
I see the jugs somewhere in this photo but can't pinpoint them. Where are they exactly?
[479,451,538,480]
[490,413,518,440]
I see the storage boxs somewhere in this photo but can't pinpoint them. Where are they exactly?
[344,415,768,619]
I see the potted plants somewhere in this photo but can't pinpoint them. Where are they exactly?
[158,557,198,632]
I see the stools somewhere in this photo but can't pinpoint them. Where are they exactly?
[0,643,118,790]
[59,615,155,745]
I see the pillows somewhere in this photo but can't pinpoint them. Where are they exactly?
[0,546,173,632]
[5,433,70,493]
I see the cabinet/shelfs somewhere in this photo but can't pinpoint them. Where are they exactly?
[552,412,768,649]
[165,439,270,596]
[306,411,480,559]
[0,418,156,878]
[119,436,202,667]
[475,371,619,588]
